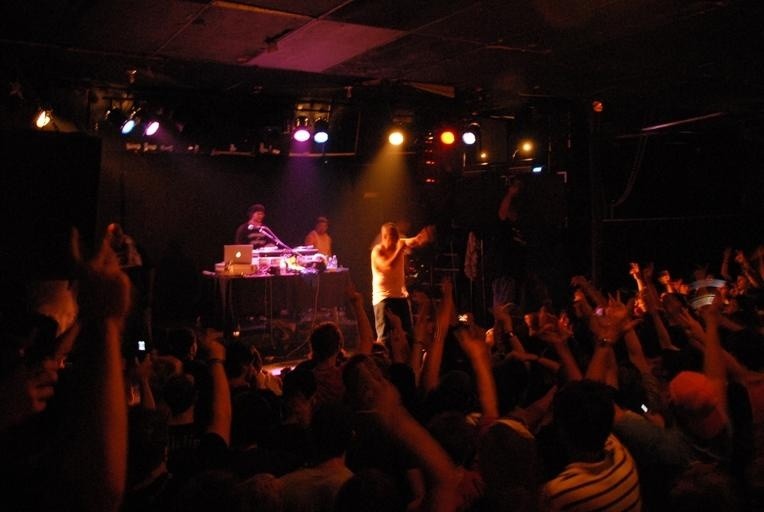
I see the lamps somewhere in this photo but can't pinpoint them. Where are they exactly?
[291,114,329,145]
[461,122,480,145]
[103,104,160,137]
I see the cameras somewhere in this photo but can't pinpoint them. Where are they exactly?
[456,313,469,323]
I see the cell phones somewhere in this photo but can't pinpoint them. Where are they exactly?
[137,339,147,363]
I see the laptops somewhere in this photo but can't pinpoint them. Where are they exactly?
[215,244,253,266]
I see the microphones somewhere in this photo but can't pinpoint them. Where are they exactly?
[247,224,265,230]
